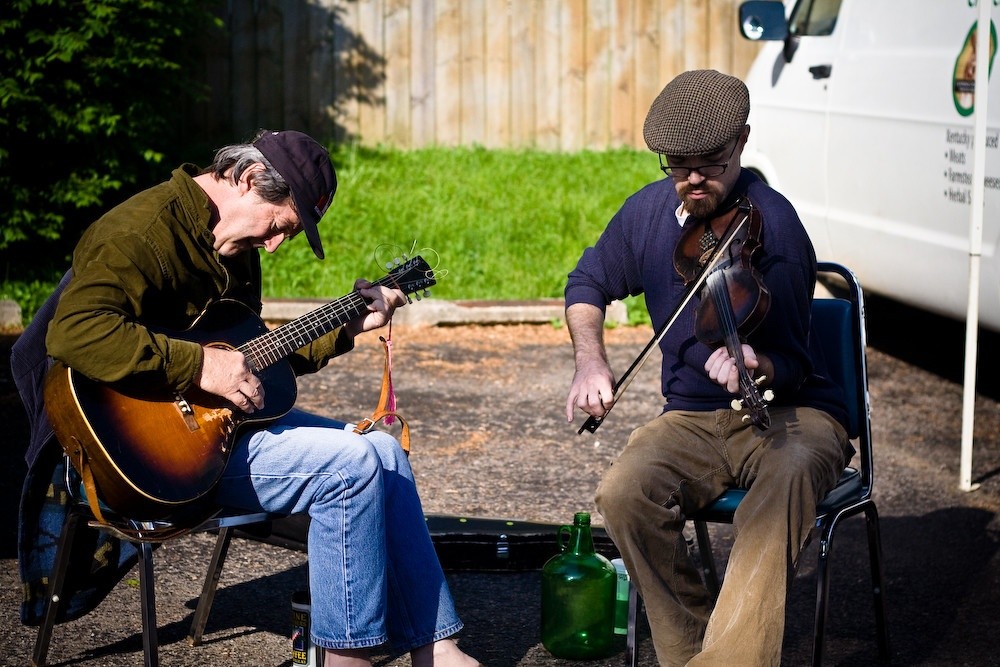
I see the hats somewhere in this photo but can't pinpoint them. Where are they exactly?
[643,69,750,156]
[251,129,337,260]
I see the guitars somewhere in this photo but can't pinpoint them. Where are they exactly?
[42,253,436,542]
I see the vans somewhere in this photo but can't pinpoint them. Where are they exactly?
[736,1,1000,330]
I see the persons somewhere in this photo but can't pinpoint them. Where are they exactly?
[565,69,857,667]
[46,131,481,667]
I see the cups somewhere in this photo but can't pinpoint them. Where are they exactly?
[609,558,631,635]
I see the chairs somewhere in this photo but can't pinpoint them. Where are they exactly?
[33,455,311,667]
[627,263,884,667]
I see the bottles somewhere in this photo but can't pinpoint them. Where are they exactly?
[290,565,316,667]
[539,512,618,661]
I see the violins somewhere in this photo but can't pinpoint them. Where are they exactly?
[672,194,774,433]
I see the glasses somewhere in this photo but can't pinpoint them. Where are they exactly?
[658,133,741,179]
